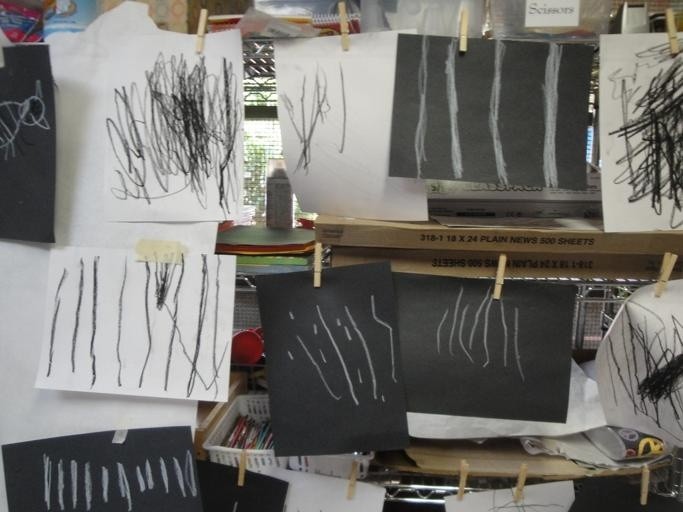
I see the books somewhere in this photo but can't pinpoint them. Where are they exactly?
[230,253,315,266]
[215,224,317,256]
